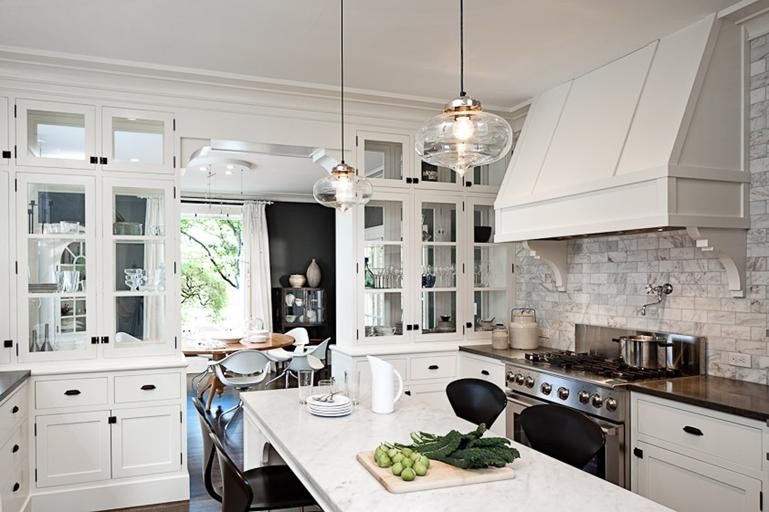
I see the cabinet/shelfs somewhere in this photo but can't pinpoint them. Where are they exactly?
[353,129,509,356]
[32,343,191,512]
[459,344,506,439]
[179,109,359,199]
[16,98,176,362]
[0,370,31,512]
[328,341,459,415]
[280,288,326,336]
[626,375,769,512]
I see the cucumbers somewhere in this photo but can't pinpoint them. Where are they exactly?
[419,422,486,438]
[465,438,512,447]
[442,445,521,469]
[422,431,461,460]
[418,435,476,442]
[409,432,423,445]
[394,430,455,451]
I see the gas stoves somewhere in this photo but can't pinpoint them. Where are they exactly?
[503,324,709,424]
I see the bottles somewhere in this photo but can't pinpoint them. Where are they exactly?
[30,330,40,352]
[41,324,53,351]
[422,265,436,288]
[365,258,375,287]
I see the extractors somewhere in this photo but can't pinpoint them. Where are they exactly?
[489,18,747,242]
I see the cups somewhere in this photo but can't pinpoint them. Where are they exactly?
[474,264,482,286]
[295,298,302,306]
[344,366,362,408]
[55,271,65,291]
[64,270,80,292]
[366,355,405,416]
[371,265,403,289]
[423,264,454,287]
[317,380,334,396]
[297,368,315,407]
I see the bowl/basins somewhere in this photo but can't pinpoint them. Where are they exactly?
[440,314,451,322]
[286,315,296,323]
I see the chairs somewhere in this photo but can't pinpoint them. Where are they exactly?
[209,433,319,512]
[446,378,508,430]
[521,405,606,470]
[267,337,331,389]
[280,327,310,369]
[239,384,678,512]
[191,397,230,503]
[205,349,272,430]
[263,348,293,373]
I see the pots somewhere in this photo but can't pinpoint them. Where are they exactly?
[610,332,687,372]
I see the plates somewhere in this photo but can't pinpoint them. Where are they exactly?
[210,338,246,344]
[303,398,354,418]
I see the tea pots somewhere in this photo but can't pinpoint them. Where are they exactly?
[374,325,397,336]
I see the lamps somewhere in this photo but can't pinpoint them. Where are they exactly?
[413,0,513,180]
[312,0,373,216]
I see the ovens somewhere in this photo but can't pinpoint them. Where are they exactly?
[506,390,631,491]
